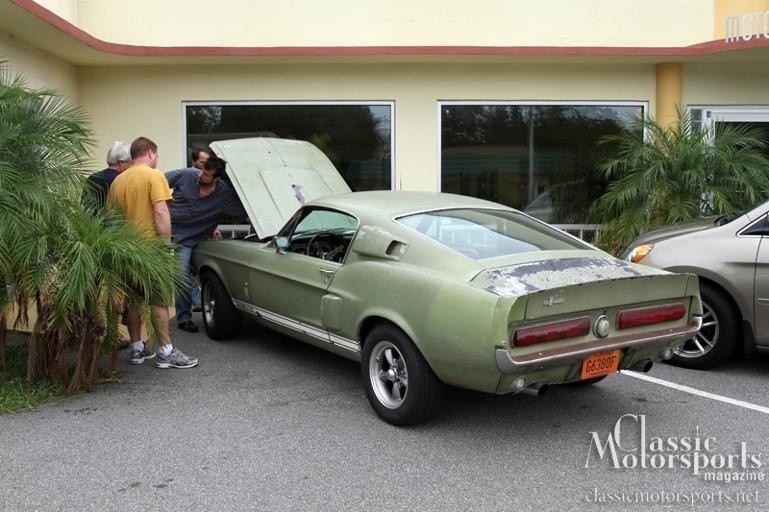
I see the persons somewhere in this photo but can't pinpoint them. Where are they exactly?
[188,149,213,312]
[81,140,133,351]
[160,156,248,334]
[104,136,199,370]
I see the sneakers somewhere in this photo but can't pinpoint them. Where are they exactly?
[178,320,198,333]
[155,346,199,369]
[130,341,156,364]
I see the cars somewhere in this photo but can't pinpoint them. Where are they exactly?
[617,198,769,370]
[189,137,704,427]
